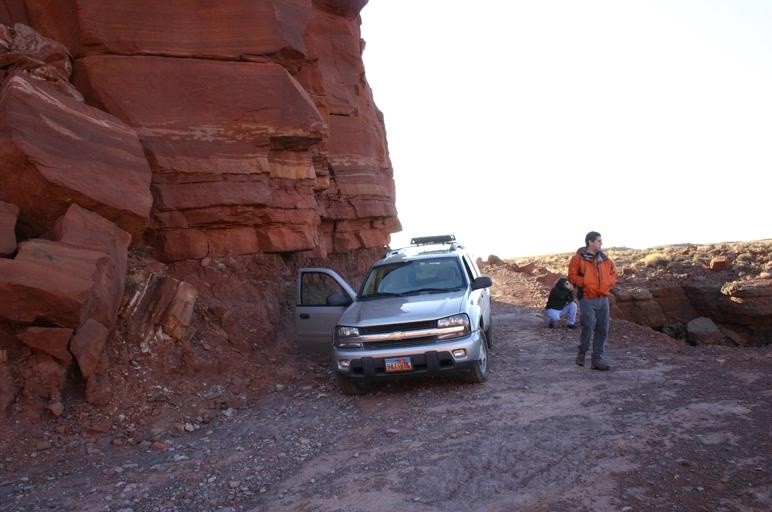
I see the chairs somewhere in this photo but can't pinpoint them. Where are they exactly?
[383,266,456,293]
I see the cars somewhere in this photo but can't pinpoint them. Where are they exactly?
[295,234,492,396]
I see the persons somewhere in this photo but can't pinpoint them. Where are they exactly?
[546,278,577,329]
[568,231,618,370]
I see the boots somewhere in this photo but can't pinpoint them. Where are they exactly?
[591,360,610,370]
[550,321,576,328]
[576,352,585,366]
